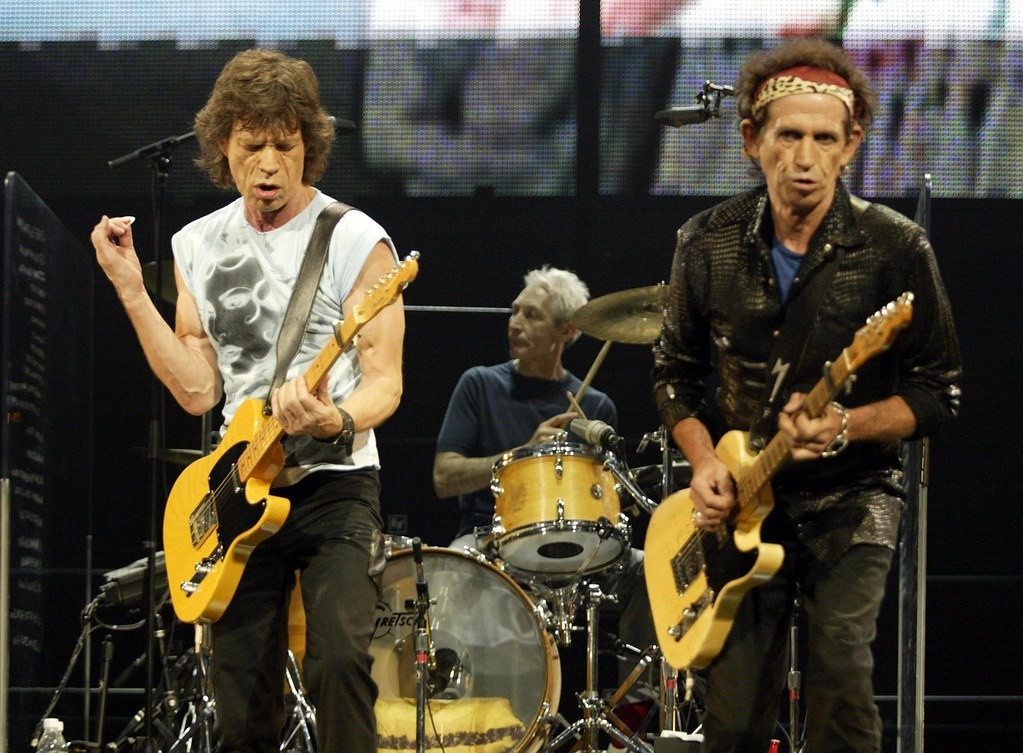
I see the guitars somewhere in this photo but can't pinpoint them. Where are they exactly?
[644,290,916,671]
[161,247,422,627]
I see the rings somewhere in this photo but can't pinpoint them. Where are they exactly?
[697,512,701,518]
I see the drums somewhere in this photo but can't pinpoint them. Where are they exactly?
[369,543,564,753]
[284,534,428,707]
[493,442,627,579]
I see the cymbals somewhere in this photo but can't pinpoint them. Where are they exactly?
[570,285,668,345]
[162,448,214,465]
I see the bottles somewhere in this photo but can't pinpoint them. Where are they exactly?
[37,718,64,753]
[54,721,68,753]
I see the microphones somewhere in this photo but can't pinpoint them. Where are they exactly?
[100,550,169,609]
[570,418,624,447]
[330,116,357,144]
[427,648,457,694]
[653,107,719,128]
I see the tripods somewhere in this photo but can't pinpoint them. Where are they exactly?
[538,450,702,753]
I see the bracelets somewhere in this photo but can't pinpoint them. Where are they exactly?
[310,409,355,457]
[822,403,848,457]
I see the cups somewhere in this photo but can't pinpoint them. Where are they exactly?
[768,740,780,753]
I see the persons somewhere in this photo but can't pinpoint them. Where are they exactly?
[433,270,660,685]
[653,39,961,753]
[92,50,405,753]
